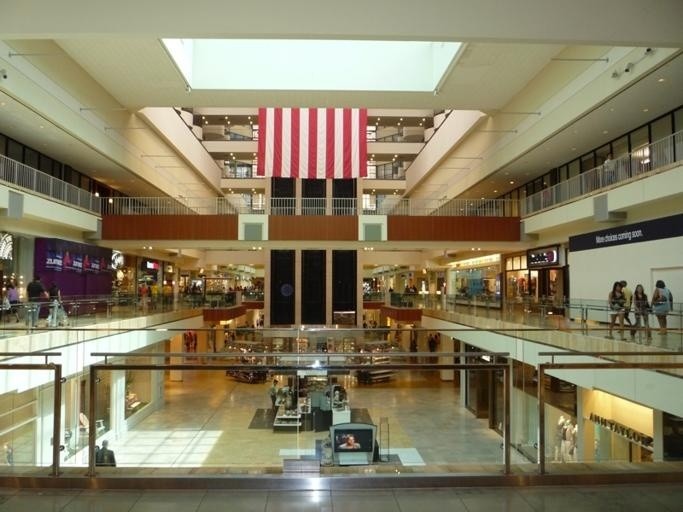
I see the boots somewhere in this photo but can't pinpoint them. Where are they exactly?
[657,328,668,335]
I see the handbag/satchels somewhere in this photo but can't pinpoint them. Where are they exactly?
[653,295,667,306]
[2,296,11,310]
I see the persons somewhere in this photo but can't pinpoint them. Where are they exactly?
[554,415,578,462]
[630,284,653,341]
[403,278,417,294]
[651,280,673,336]
[95,439,116,467]
[6,285,20,323]
[607,280,633,341]
[338,433,361,449]
[26,275,63,328]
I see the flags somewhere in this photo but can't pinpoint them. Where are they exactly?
[256,108,368,179]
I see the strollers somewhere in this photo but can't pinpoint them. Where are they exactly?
[54,301,70,327]
